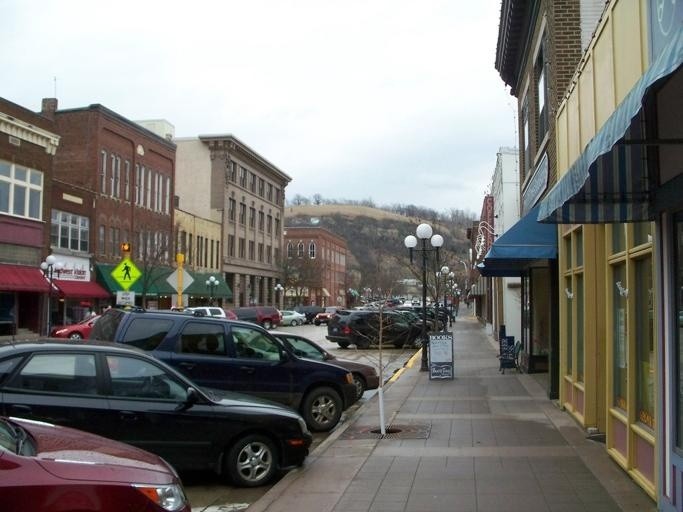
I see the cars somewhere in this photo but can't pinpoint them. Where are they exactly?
[0,338,312,489]
[326,296,456,350]
[0,416,191,512]
[84,309,358,431]
[50,314,101,343]
[183,305,334,331]
[201,330,378,400]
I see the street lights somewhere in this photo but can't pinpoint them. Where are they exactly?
[42,256,62,337]
[404,223,461,372]
[274,283,284,306]
[205,276,220,306]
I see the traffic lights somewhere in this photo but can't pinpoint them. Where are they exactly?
[122,242,130,254]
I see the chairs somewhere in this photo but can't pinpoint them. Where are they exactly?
[497,340,522,374]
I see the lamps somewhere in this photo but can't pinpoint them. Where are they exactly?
[616,281,629,299]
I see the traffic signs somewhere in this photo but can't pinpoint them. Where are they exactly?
[115,291,134,306]
